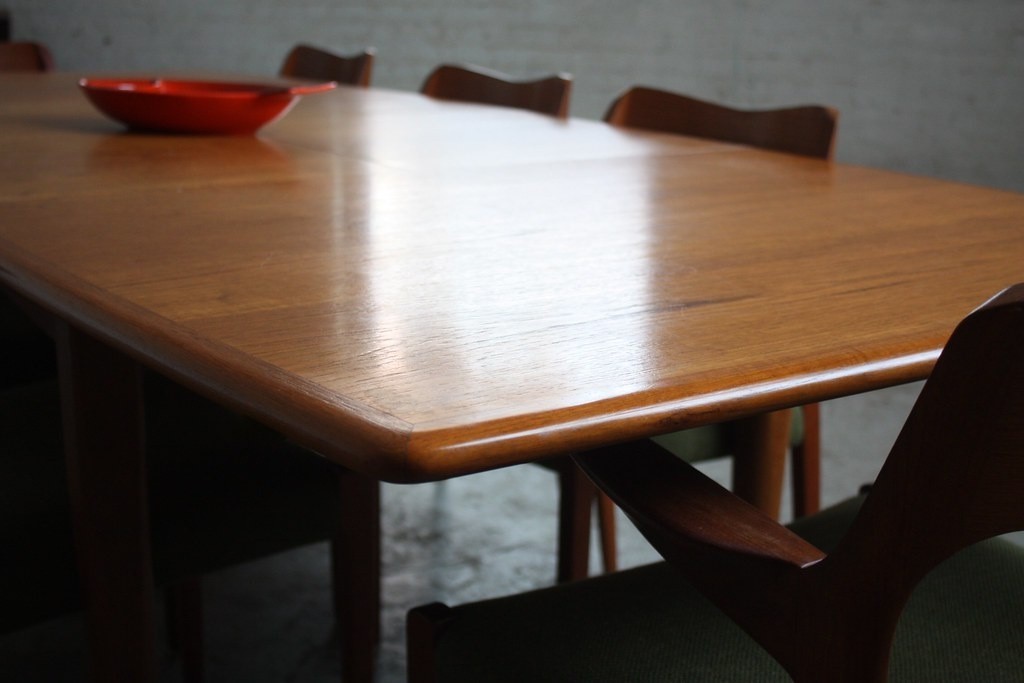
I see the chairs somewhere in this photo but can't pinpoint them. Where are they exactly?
[0,40,1024,683]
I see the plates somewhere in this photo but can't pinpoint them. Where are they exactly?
[78,76,302,135]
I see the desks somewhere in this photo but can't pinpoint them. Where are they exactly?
[0,71,1024,683]
